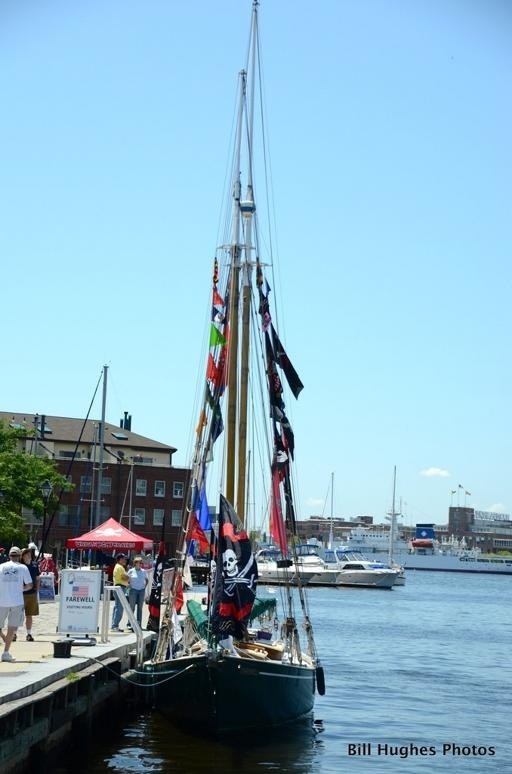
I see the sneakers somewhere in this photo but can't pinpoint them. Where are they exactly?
[26,635,34,641]
[12,634,17,641]
[1,652,16,662]
[111,624,133,632]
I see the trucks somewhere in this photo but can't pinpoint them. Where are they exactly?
[163,558,210,584]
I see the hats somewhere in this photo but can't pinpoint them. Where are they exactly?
[9,546,32,557]
[28,542,38,550]
[115,553,128,561]
[133,557,143,564]
[0,548,6,552]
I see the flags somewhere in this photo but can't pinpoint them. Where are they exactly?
[146,515,165,634]
[212,494,259,640]
[256,257,305,556]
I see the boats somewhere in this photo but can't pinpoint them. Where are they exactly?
[254,461,511,592]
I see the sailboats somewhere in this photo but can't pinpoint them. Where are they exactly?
[123,0,329,735]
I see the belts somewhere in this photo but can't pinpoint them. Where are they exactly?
[114,583,127,588]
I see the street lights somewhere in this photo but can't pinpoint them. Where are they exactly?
[40,478,52,563]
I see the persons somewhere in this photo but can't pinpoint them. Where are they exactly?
[0,546,149,661]
